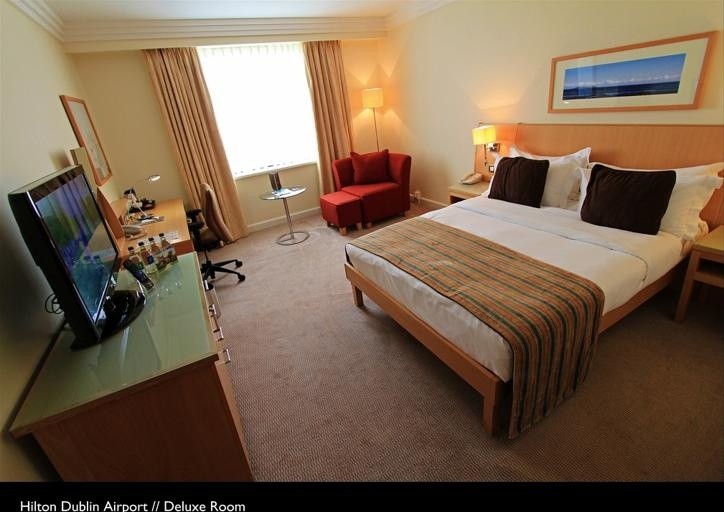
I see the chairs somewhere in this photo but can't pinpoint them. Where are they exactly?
[186,183,245,290]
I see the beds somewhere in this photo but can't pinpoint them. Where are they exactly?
[345,123,724,441]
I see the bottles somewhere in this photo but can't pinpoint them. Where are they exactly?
[128,233,178,276]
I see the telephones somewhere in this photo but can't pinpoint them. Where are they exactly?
[121,225,142,234]
[461,173,482,184]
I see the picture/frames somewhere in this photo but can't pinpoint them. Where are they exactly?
[547,31,718,114]
[59,94,113,186]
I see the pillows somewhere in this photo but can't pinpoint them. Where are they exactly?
[488,156,550,209]
[482,154,577,208]
[509,147,592,196]
[577,168,724,239]
[579,165,676,236]
[349,149,390,185]
[588,162,724,235]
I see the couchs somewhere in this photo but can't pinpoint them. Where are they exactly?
[331,154,411,228]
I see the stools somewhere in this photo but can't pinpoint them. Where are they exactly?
[319,192,363,236]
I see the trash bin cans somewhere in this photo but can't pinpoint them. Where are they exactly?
[204,283,222,319]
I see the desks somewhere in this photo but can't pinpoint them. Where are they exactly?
[109,196,196,269]
[260,185,310,246]
[9,250,255,482]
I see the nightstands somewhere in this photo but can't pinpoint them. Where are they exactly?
[446,180,490,203]
[674,225,724,324]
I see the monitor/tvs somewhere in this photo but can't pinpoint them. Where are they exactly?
[9,163,122,344]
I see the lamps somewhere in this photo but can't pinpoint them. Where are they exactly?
[129,175,161,220]
[472,124,499,167]
[363,88,383,152]
